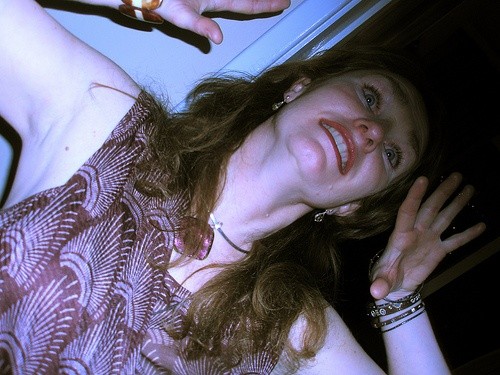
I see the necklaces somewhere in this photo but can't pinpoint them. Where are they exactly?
[173,183,250,260]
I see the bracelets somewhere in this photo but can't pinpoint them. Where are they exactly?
[118,0,163,24]
[368,247,426,335]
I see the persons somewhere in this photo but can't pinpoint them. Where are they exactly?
[0,1,486,375]
